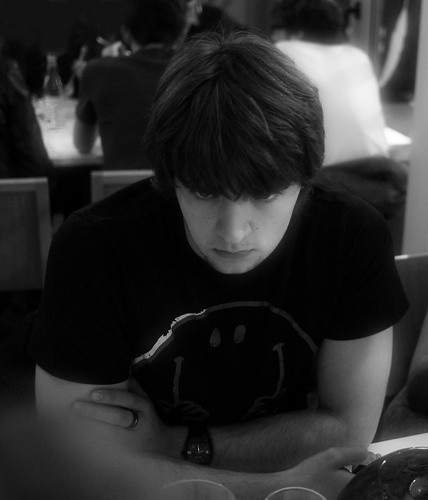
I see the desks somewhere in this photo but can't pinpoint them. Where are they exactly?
[31,95,412,166]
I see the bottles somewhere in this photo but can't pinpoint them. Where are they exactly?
[43,55,63,131]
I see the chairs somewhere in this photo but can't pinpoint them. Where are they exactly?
[0,156,428,419]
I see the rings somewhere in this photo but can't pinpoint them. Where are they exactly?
[126,413,139,431]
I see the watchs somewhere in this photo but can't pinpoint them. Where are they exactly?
[181,422,213,465]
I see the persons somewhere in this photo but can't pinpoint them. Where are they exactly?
[265,0,407,257]
[0,55,56,200]
[31,31,411,500]
[74,0,180,170]
